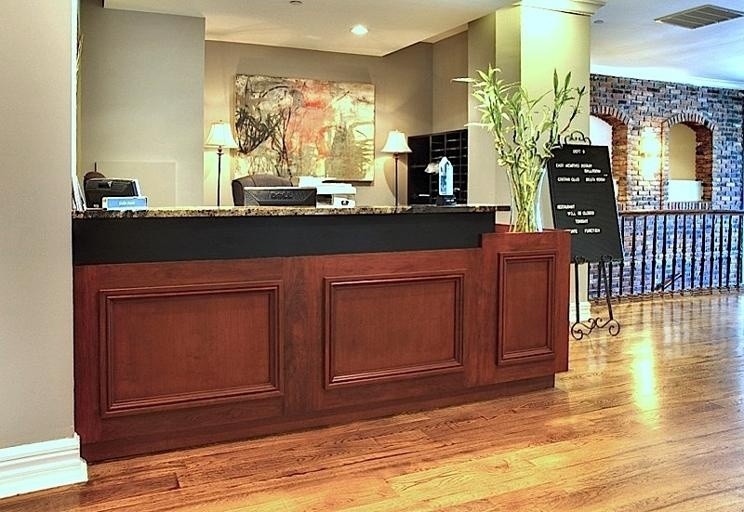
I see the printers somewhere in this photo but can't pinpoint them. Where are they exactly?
[291,176,356,209]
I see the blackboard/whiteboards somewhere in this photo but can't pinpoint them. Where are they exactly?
[546,144,624,264]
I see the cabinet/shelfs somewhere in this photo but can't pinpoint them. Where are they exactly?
[407,129,468,205]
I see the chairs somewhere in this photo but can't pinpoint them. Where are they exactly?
[232,174,293,206]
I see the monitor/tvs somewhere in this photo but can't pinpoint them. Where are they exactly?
[84,178,141,209]
[244,186,317,207]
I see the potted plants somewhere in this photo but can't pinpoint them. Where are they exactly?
[449,62,589,232]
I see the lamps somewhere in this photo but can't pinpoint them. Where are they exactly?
[204,120,238,206]
[381,129,413,206]
